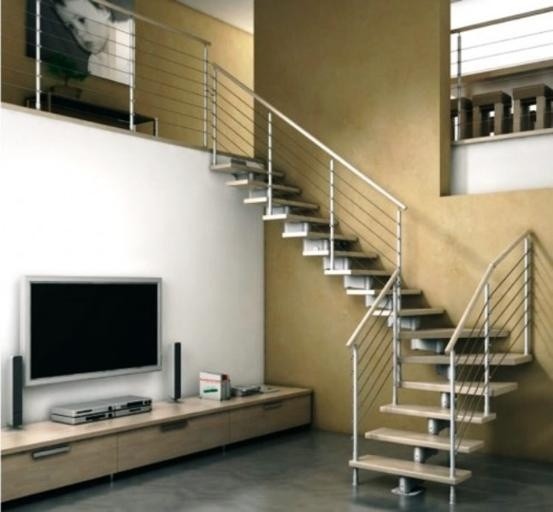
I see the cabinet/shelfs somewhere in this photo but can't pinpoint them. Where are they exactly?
[2,396,312,500]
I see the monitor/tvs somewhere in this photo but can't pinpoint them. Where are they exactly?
[20,274,163,386]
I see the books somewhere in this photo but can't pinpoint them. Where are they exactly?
[231,385,261,396]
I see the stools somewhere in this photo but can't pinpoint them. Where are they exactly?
[450,79,553,139]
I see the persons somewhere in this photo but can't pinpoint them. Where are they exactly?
[53,0,136,88]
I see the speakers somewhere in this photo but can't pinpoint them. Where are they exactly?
[171,342,182,402]
[10,355,22,426]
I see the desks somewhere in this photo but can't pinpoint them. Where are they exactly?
[24,93,157,140]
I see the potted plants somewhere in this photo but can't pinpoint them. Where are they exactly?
[41,27,93,99]
